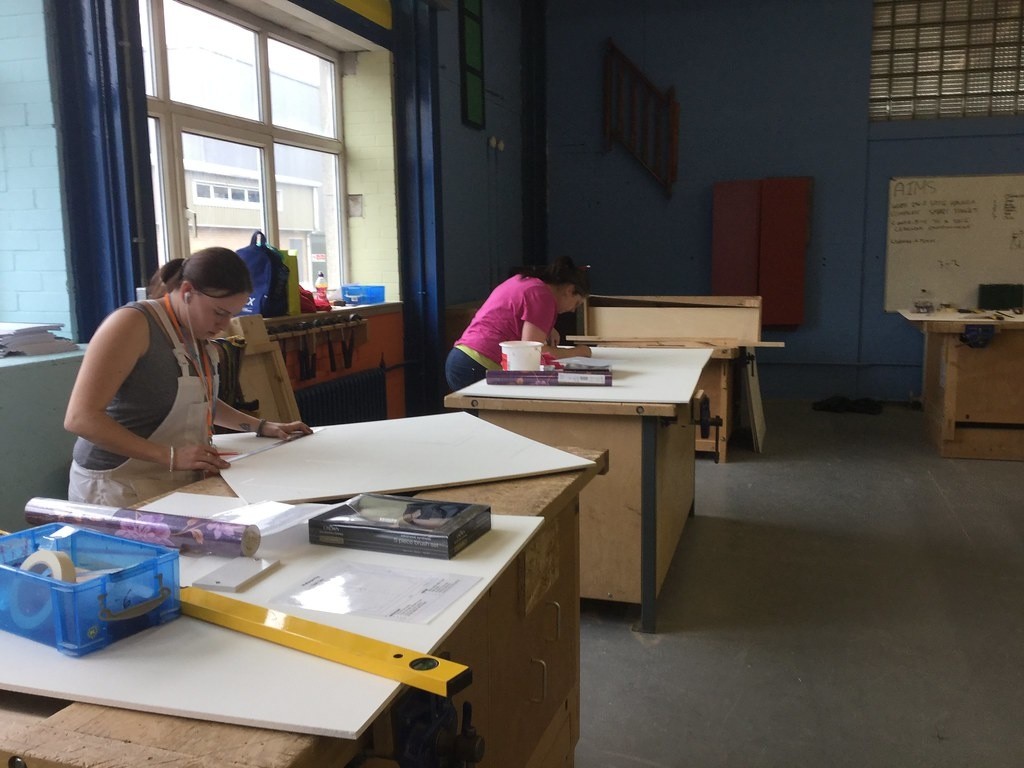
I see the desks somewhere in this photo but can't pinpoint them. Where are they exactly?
[445,349,712,633]
[899,307,1024,460]
[0,445,610,768]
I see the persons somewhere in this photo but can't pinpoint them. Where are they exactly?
[63,247,313,507]
[445,257,592,392]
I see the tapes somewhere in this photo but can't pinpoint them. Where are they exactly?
[9,549,75,630]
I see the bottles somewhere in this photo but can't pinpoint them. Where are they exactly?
[315,271,327,301]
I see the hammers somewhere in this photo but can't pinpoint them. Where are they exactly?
[264,313,363,382]
[329,299,346,306]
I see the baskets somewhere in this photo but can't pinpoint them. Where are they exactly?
[342,285,386,305]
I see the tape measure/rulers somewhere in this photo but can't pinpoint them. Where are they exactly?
[221,431,313,462]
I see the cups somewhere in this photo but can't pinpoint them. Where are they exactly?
[499,340,545,370]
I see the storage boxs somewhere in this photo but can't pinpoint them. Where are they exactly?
[0,523,182,656]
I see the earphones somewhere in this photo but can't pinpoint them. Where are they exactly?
[185,292,191,304]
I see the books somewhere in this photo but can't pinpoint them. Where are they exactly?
[0,322,80,359]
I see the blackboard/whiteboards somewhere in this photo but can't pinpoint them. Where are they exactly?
[885,174,1024,312]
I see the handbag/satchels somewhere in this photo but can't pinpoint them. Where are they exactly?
[235,230,288,317]
[279,249,301,315]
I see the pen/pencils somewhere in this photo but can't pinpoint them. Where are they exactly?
[218,451,240,455]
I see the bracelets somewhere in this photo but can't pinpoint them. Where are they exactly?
[169,445,174,473]
[256,419,267,437]
